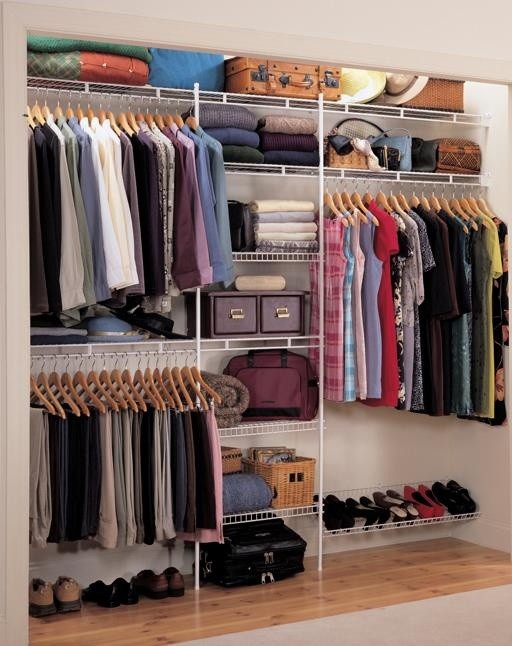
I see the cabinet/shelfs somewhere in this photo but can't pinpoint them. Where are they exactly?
[27,76,488,591]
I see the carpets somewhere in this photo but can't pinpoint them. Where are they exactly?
[166,582,512,646]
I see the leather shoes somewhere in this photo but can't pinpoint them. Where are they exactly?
[314,479,477,535]
[29,567,185,618]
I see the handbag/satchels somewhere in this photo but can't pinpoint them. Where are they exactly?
[223,350,320,421]
[227,199,254,252]
[326,127,482,176]
[198,513,306,586]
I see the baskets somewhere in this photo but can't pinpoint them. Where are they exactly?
[220,446,243,474]
[241,455,316,508]
[403,79,465,112]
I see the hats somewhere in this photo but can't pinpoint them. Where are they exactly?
[86,309,194,341]
[340,68,429,106]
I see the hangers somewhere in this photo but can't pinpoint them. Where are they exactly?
[26,87,198,137]
[314,176,499,235]
[29,347,222,419]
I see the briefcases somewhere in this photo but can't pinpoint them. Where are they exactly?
[224,55,342,102]
